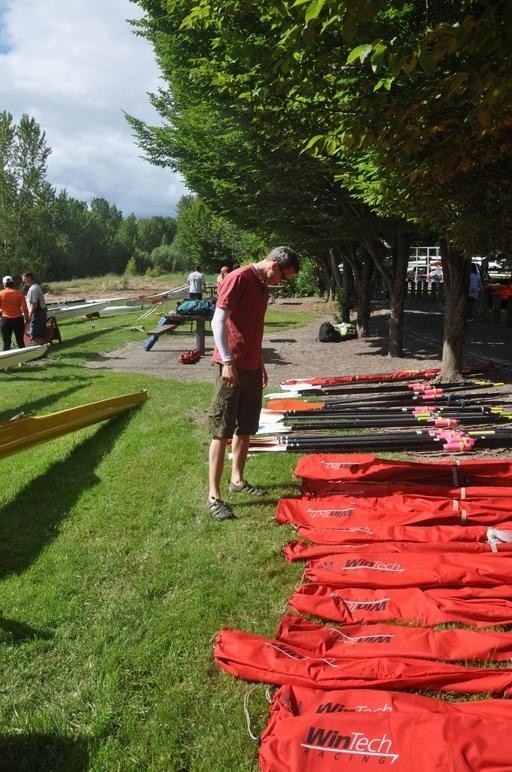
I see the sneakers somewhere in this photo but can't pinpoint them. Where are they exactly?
[229,480,268,496]
[208,497,234,521]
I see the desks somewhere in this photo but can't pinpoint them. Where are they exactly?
[206,286,218,297]
[268,285,284,303]
[166,313,212,354]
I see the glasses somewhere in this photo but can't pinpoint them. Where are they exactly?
[278,264,288,280]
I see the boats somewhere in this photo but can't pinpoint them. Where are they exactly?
[18,299,109,320]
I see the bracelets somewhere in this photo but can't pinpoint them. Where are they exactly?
[222,356,234,365]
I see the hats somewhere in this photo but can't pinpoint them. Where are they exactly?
[2,276,13,283]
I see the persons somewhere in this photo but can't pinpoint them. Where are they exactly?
[467,263,483,321]
[206,247,298,521]
[187,265,205,300]
[22,273,46,345]
[217,264,241,286]
[0,275,30,351]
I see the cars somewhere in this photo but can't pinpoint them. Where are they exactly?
[408,266,431,281]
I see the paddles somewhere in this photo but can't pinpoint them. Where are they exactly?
[224,375,511,454]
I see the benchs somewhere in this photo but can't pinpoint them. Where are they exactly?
[147,324,179,338]
